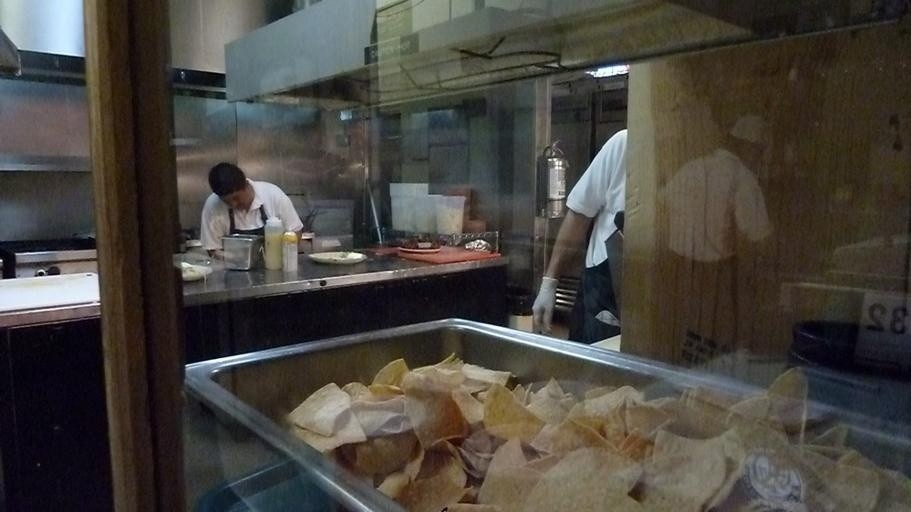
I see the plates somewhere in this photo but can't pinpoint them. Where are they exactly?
[183,263,213,282]
[396,246,442,254]
[308,251,368,264]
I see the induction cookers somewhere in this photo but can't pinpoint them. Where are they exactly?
[2,237,98,257]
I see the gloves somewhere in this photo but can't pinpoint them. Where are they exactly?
[532,277,559,332]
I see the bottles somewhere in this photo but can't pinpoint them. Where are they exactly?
[263,216,299,272]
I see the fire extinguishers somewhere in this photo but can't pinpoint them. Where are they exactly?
[537,137,568,220]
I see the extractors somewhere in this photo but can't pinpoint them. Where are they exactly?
[0,67,91,171]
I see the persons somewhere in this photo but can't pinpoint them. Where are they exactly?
[200,160,305,269]
[532,125,628,344]
[648,113,776,354]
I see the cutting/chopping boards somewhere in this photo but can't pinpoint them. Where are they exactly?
[0,271,102,313]
[370,245,503,264]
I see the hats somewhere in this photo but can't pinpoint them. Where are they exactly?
[728,114,768,146]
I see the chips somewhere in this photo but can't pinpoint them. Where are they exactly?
[287,350,895,512]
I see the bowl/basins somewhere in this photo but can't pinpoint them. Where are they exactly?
[792,322,859,361]
